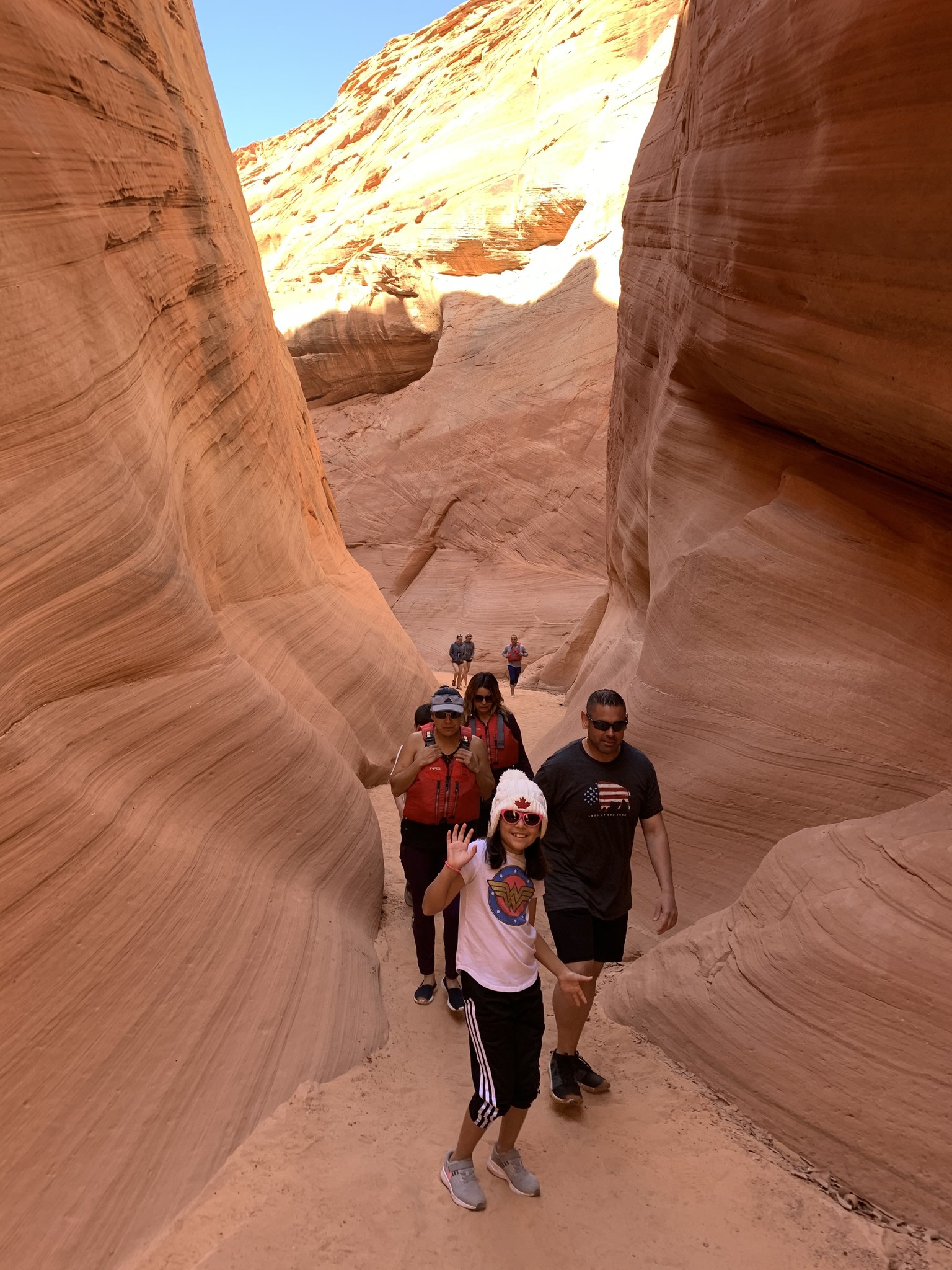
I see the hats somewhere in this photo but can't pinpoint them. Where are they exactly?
[466,633,473,639]
[430,694,464,713]
[487,769,548,840]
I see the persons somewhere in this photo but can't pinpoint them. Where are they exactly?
[526,689,678,1107]
[449,633,475,689]
[386,671,533,1012]
[502,635,529,698]
[421,770,592,1211]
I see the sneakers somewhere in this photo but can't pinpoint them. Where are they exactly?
[440,1150,487,1211]
[568,1050,608,1094]
[413,979,438,1005]
[440,975,465,1012]
[486,1142,540,1197]
[548,1048,584,1106]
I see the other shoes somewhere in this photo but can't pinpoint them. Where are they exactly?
[464,685,468,688]
[460,685,463,686]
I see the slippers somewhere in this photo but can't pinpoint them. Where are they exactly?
[404,882,413,907]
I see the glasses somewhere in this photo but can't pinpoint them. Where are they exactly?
[466,635,473,638]
[433,711,461,719]
[586,709,628,732]
[473,695,493,703]
[501,809,544,827]
[458,635,463,636]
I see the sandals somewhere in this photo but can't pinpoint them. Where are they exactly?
[452,680,456,687]
[456,685,462,690]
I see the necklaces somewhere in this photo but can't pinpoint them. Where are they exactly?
[585,737,591,756]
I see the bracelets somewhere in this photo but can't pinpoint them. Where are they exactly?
[445,861,461,872]
